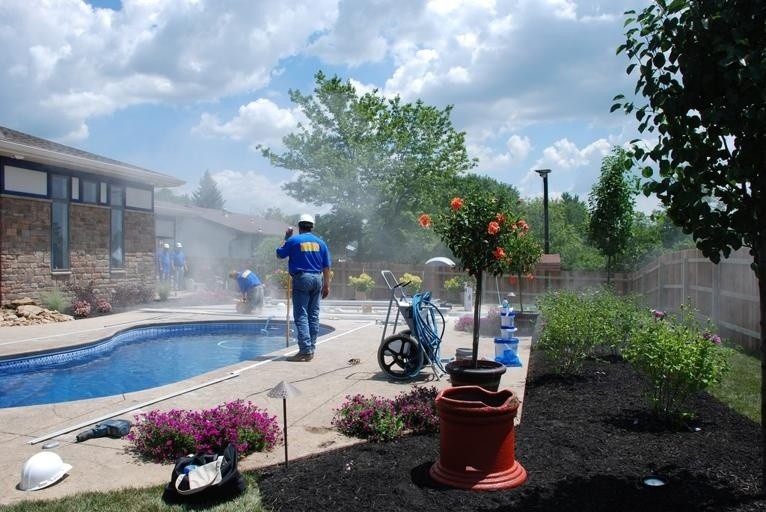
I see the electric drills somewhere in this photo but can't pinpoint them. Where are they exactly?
[63,420,132,445]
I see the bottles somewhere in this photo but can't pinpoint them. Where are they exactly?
[183,464,200,476]
[501,297,509,314]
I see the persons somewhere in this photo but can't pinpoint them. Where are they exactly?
[158,243,170,285]
[277,213,330,363]
[170,241,189,292]
[227,268,263,313]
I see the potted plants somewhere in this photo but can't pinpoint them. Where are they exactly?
[340,272,463,299]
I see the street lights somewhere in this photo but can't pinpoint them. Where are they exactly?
[535,169,552,289]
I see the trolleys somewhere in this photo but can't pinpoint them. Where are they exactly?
[377,271,447,381]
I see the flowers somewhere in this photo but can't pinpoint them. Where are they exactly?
[420,194,532,364]
[267,269,292,287]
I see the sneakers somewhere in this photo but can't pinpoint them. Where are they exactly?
[287,350,314,361]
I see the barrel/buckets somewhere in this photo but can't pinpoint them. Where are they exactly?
[493,311,520,362]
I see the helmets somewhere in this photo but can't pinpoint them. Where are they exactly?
[176,243,182,248]
[163,244,169,248]
[297,213,314,224]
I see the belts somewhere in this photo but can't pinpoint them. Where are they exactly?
[304,271,322,274]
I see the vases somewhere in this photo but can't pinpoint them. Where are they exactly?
[512,309,537,334]
[430,385,528,492]
[276,287,292,299]
[446,357,506,391]
